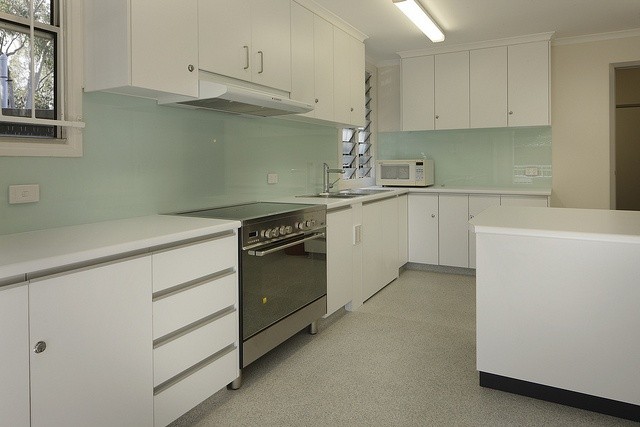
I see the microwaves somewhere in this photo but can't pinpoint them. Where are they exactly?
[375,158,434,187]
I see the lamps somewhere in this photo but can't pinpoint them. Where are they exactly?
[393,0,446,44]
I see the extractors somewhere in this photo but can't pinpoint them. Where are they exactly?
[156,79,315,118]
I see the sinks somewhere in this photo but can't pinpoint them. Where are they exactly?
[342,188,393,195]
[296,194,359,198]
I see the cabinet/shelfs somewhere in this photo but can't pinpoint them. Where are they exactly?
[407,193,469,269]
[290,0,367,131]
[360,198,397,303]
[470,40,551,129]
[81,1,199,101]
[326,204,358,318]
[157,1,314,119]
[400,50,470,130]
[470,194,549,270]
[0,251,154,427]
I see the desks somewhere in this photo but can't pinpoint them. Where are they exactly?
[472,204,640,422]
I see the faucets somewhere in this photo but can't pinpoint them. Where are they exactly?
[323,162,346,192]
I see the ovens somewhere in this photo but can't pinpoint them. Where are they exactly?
[158,202,326,367]
